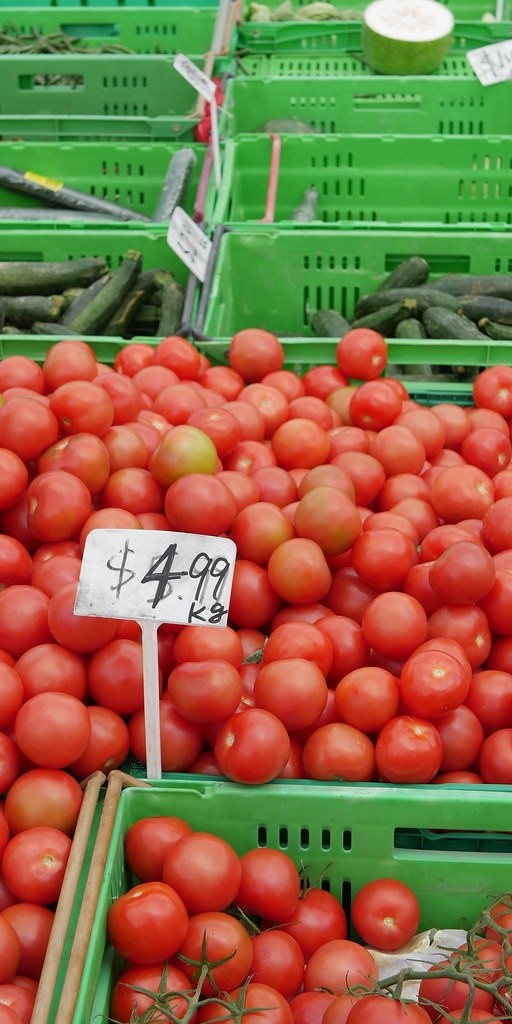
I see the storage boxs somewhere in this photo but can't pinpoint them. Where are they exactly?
[228,75,512,142]
[0,229,201,369]
[235,0,512,51]
[238,53,483,132]
[0,6,239,90]
[202,228,512,401]
[213,135,512,315]
[46,778,512,1024]
[0,142,223,262]
[0,54,229,142]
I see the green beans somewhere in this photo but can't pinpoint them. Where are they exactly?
[0,20,141,89]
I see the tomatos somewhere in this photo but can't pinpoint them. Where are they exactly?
[0,322,512,1024]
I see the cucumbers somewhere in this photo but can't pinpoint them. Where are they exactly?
[307,256,512,381]
[0,249,185,335]
[0,146,198,219]
